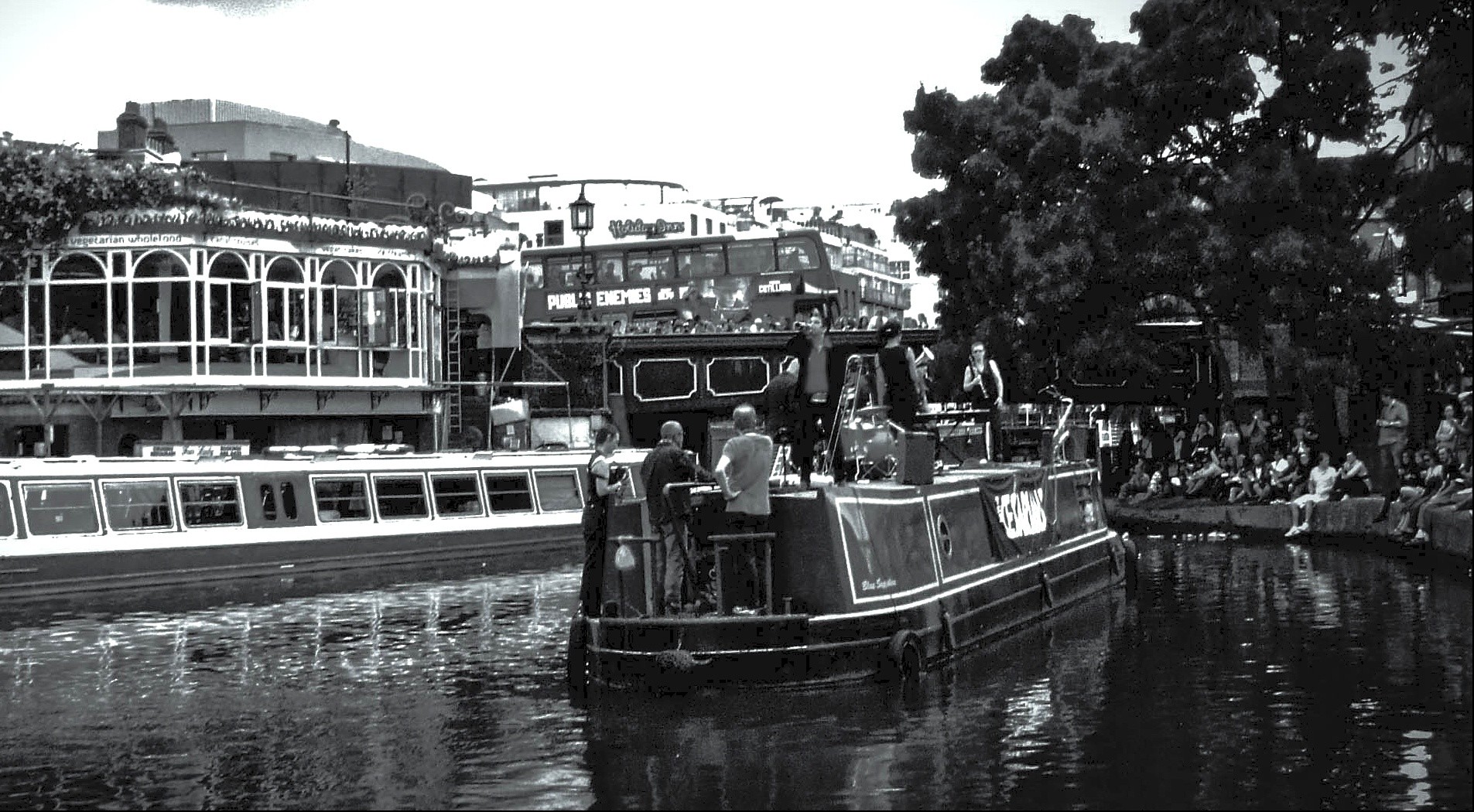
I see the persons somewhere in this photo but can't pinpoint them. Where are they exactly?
[211,312,334,363]
[613,320,621,334]
[640,421,716,616]
[633,308,942,334]
[715,403,773,614]
[682,276,745,320]
[1434,350,1473,392]
[763,359,821,473]
[61,327,123,364]
[785,314,847,486]
[874,320,923,430]
[1115,385,1472,549]
[579,424,630,620]
[574,262,642,284]
[963,342,1004,462]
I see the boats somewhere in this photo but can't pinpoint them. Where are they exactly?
[0,441,700,632]
[566,352,1127,701]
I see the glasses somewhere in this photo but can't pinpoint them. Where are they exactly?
[972,350,985,353]
[676,432,686,436]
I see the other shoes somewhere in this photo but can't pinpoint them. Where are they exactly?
[797,479,810,492]
[1297,521,1310,532]
[732,605,758,615]
[666,604,694,619]
[1387,527,1431,548]
[1285,525,1301,537]
[654,604,665,618]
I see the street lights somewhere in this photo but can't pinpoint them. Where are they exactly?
[569,190,596,322]
